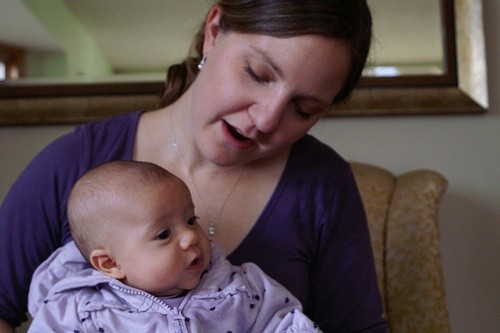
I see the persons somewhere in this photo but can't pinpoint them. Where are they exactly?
[0,0,392,333]
[26,160,323,333]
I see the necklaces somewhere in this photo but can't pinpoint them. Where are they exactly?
[168,104,249,236]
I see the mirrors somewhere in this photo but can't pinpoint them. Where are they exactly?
[0,1,490,128]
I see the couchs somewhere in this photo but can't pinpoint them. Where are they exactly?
[348,162,451,333]
